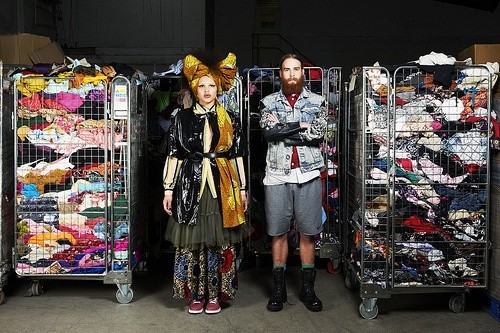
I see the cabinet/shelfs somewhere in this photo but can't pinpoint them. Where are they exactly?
[12,62,490,320]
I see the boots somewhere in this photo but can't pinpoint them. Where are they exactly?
[298,267,323,312]
[268,267,295,312]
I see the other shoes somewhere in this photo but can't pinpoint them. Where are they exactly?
[189,298,204,314]
[205,298,221,314]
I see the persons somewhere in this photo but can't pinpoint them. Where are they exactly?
[163,55,247,314]
[258,54,327,313]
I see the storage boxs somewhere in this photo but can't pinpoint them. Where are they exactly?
[0,34,64,66]
[458,44,500,93]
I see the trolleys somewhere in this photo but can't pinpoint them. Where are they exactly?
[10,72,146,304]
[143,73,244,276]
[341,60,493,320]
[243,66,344,276]
